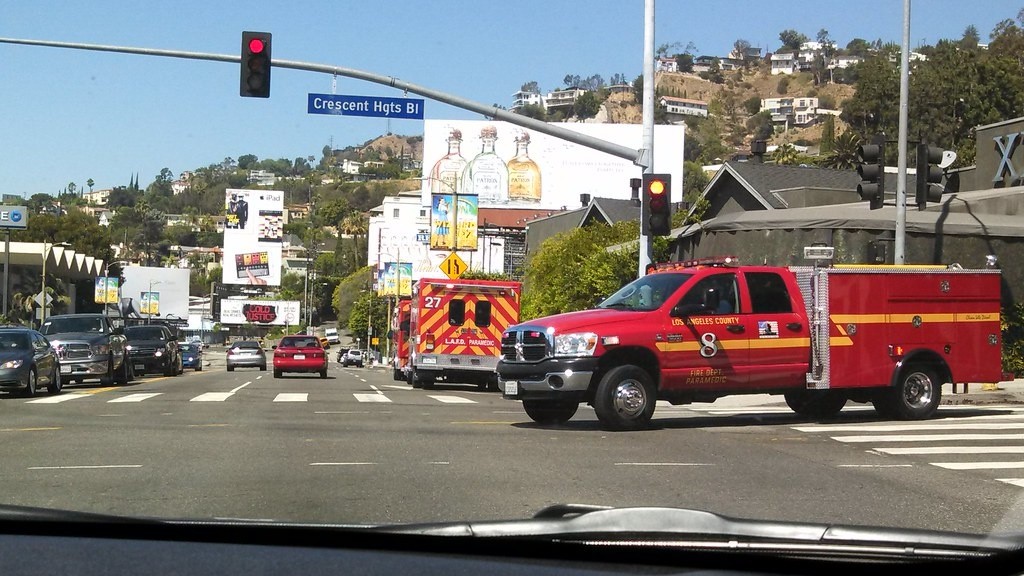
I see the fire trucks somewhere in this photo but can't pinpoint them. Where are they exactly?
[392,277,522,391]
[496,242,1016,431]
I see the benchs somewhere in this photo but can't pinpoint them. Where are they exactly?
[287,343,315,346]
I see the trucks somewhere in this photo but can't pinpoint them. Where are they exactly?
[325,328,341,344]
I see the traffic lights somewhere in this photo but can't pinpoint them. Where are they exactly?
[239,31,272,98]
[915,144,943,211]
[642,174,671,235]
[857,135,885,210]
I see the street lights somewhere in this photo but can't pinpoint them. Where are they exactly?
[148,280,167,323]
[41,239,72,325]
[201,292,219,344]
[104,260,128,314]
[412,177,460,253]
[376,247,409,306]
[360,288,372,364]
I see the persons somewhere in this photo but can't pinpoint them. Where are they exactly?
[225,194,248,229]
[434,198,449,246]
[246,269,268,286]
[379,272,384,295]
[387,265,395,294]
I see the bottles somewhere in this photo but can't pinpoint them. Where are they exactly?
[461,126,509,207]
[429,130,469,191]
[506,129,541,205]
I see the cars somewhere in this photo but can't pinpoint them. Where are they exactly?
[123,324,210,377]
[271,335,330,379]
[320,337,330,349]
[225,340,269,371]
[0,326,61,398]
[37,314,135,387]
[336,345,363,368]
